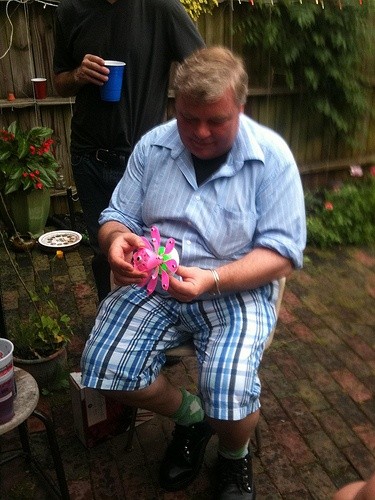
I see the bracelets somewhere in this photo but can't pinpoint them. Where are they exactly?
[212,269,219,282]
[209,268,220,296]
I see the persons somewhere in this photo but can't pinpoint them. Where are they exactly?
[80,42,307,500]
[53,0,208,367]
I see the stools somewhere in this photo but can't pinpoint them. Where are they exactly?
[1,367,69,500]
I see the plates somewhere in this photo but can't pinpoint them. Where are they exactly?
[38,230,82,248]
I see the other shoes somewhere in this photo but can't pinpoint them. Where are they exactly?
[216,443,257,500]
[158,392,216,490]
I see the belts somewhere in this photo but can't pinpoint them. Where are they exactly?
[95,147,131,169]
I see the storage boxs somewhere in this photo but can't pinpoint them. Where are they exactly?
[68,370,124,450]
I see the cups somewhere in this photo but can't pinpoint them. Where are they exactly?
[0,338,17,425]
[30,78,47,99]
[99,60,126,102]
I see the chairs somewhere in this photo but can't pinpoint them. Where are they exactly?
[111,267,286,457]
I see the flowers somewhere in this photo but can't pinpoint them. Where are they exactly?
[0,119,61,194]
[8,250,74,359]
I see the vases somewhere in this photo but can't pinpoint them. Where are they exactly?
[0,337,17,424]
[13,340,68,386]
[9,187,51,234]
